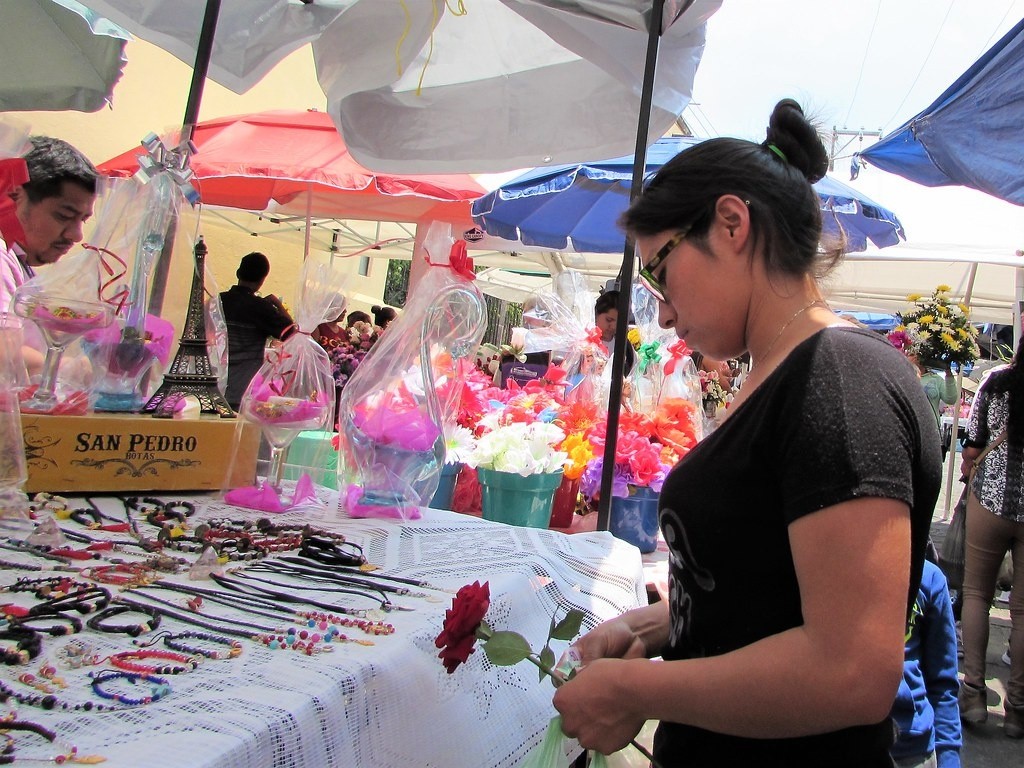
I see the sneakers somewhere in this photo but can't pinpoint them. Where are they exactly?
[954,619,964,657]
[1002,648,1011,665]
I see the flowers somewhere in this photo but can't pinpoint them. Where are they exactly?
[326,321,728,496]
[883,284,982,374]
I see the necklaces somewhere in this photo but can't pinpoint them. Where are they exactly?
[745,300,833,376]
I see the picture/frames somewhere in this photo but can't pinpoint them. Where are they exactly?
[470,483,482,515]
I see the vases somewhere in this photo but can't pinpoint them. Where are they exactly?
[428,462,463,511]
[609,484,660,554]
[451,463,479,513]
[549,475,581,528]
[478,466,564,530]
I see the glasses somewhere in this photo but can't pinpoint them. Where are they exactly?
[637,193,761,305]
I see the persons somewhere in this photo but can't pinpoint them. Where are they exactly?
[0,135,98,395]
[959,306,1024,735]
[883,555,963,768]
[585,289,638,374]
[310,292,398,355]
[550,94,942,768]
[219,251,295,413]
[906,349,957,462]
[690,347,734,395]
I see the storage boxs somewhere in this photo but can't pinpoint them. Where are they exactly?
[0,403,262,493]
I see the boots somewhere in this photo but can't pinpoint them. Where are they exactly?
[1004,696,1024,737]
[958,679,988,726]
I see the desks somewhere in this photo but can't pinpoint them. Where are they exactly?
[0,476,650,768]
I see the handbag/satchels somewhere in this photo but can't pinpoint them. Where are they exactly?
[938,483,1014,592]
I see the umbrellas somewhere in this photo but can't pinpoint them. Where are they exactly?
[92,105,490,298]
[1,0,128,115]
[470,135,906,367]
